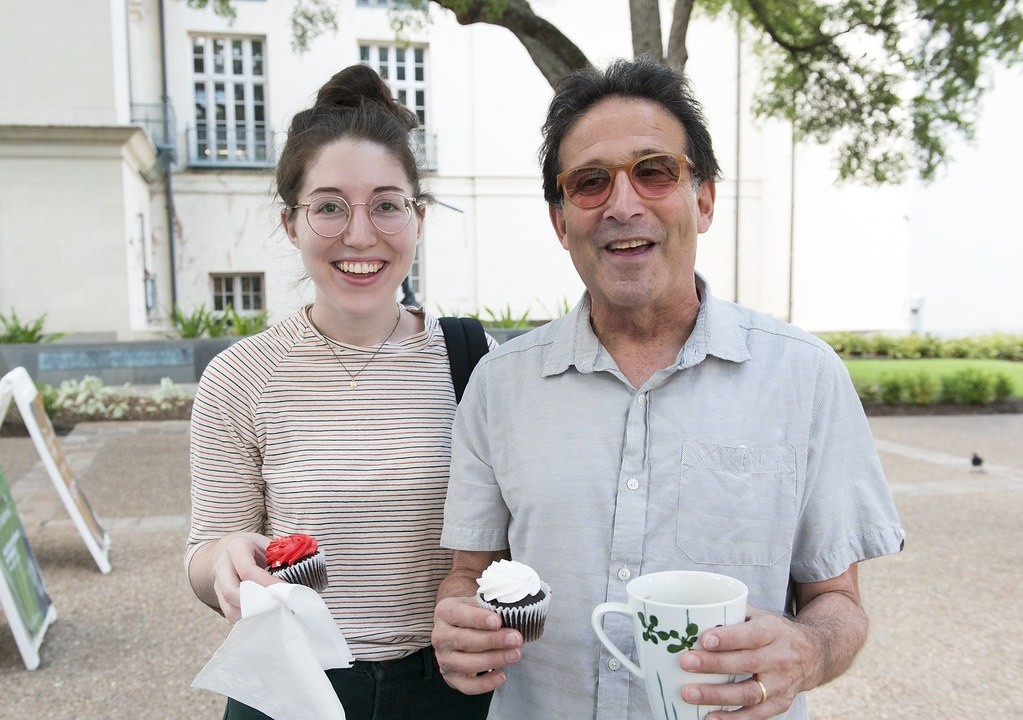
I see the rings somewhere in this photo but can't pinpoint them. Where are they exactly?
[749,675,767,704]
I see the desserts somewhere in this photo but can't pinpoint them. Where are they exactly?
[476,558,551,645]
[263,533,330,594]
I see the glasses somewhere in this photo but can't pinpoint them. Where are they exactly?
[292,191,417,239]
[556,153,699,210]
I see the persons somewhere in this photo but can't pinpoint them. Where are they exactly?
[182,64,509,720]
[432,69,913,719]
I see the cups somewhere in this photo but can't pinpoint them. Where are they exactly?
[590,571,748,720]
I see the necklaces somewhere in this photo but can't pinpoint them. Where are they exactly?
[310,300,402,388]
